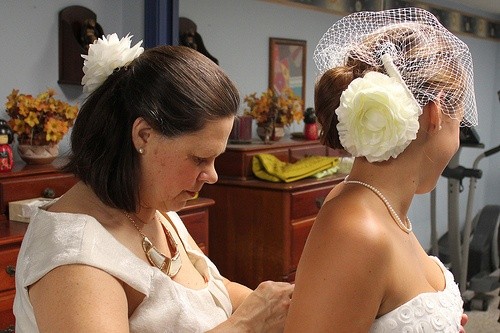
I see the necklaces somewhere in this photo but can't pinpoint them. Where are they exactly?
[341,174,414,234]
[118,206,184,278]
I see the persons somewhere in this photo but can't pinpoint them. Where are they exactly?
[16,46,472,333]
[282,18,466,333]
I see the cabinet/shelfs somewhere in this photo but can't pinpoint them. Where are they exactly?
[0,159,216,330]
[199,139,354,291]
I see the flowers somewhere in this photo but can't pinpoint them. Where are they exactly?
[5,89,79,145]
[81,33,144,99]
[334,71,421,163]
[244,85,305,129]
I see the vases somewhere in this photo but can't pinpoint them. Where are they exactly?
[257,122,285,142]
[18,134,59,166]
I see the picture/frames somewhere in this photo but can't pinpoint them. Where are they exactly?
[269,37,307,115]
